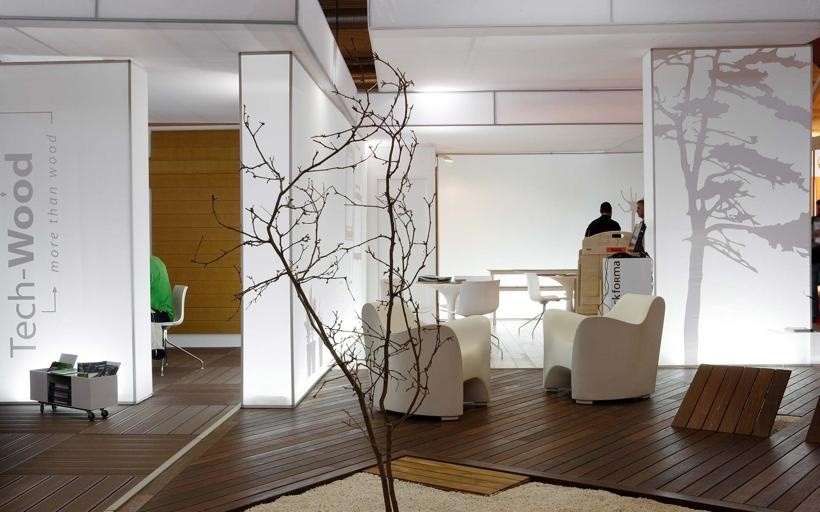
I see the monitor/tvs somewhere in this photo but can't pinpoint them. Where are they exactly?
[626,220,650,258]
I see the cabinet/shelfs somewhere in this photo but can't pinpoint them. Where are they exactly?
[31,369,118,420]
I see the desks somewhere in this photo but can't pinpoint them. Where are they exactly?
[416,274,493,321]
[488,269,577,334]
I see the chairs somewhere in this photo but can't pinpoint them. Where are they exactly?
[361,296,492,420]
[456,280,504,360]
[543,293,664,404]
[150,285,205,375]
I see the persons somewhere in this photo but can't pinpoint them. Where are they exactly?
[151,254,175,360]
[635,200,645,226]
[584,202,621,239]
[810,200,820,297]
